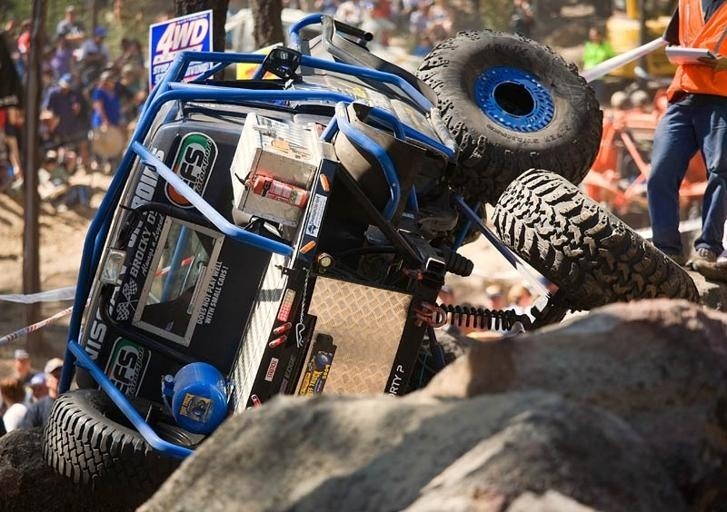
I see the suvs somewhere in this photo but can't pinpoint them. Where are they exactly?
[31,8,701,510]
[584,91,710,230]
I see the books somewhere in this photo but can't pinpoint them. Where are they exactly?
[663,47,716,66]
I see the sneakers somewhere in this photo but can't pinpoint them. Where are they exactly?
[684,247,727,281]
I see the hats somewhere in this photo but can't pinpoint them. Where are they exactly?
[44,357,64,373]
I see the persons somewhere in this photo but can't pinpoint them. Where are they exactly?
[1,347,63,433]
[693,248,727,283]
[642,1,727,271]
[0,3,150,192]
